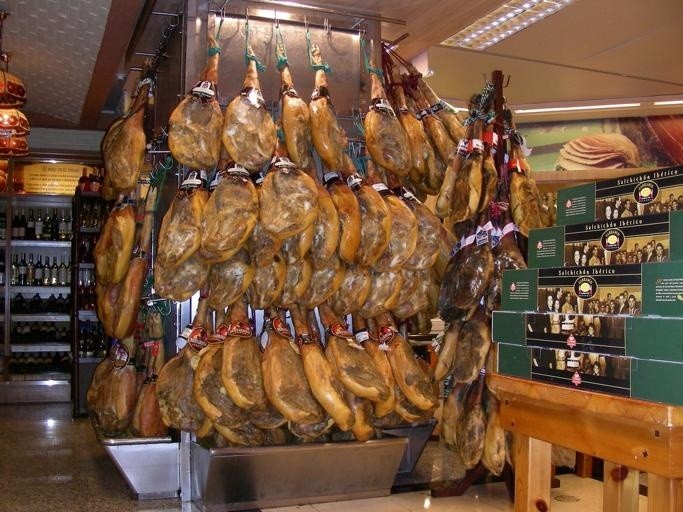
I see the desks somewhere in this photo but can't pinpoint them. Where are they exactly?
[485,373,683,512]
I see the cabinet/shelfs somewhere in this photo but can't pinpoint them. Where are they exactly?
[0,149,117,419]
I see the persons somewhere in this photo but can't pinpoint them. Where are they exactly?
[571,239,666,266]
[542,287,639,336]
[604,192,683,218]
[582,359,602,375]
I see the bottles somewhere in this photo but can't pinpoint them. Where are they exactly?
[0,208,73,241]
[80,234,96,311]
[12,321,70,344]
[9,352,69,374]
[80,201,110,227]
[0,293,71,314]
[0,253,73,287]
[79,319,106,357]
[79,166,104,192]
